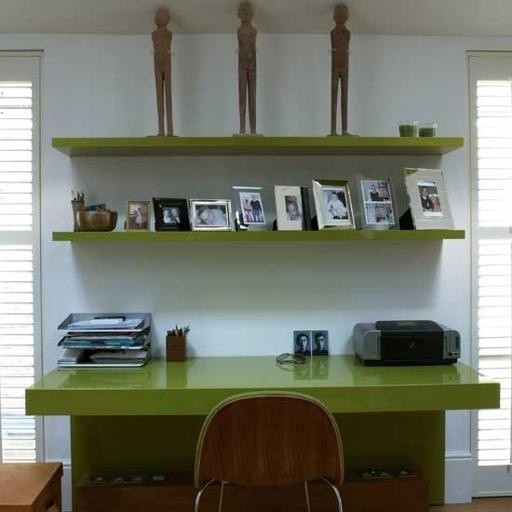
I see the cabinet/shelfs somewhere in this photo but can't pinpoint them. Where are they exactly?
[25,137,500,512]
[273,184,311,231]
[0,462,63,512]
[152,197,191,231]
[310,177,356,231]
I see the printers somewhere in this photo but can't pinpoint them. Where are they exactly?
[353,320,461,366]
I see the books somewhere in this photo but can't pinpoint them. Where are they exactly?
[61,317,150,364]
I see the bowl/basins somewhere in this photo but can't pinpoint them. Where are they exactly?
[75,209,118,232]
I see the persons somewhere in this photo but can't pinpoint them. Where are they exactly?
[164,208,180,223]
[197,209,226,224]
[313,333,328,354]
[370,185,383,201]
[245,195,262,221]
[295,334,310,356]
[288,202,302,220]
[135,206,144,225]
[374,206,387,222]
[328,192,347,218]
[422,187,434,211]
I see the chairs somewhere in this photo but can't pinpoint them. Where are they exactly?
[193,390,345,511]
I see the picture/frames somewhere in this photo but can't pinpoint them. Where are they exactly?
[188,198,233,231]
[230,185,273,231]
[124,199,150,232]
[399,167,455,230]
[354,172,400,231]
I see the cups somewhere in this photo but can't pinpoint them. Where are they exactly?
[419,120,437,138]
[396,118,419,137]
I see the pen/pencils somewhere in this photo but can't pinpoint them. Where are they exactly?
[167,324,190,336]
[70,190,86,202]
